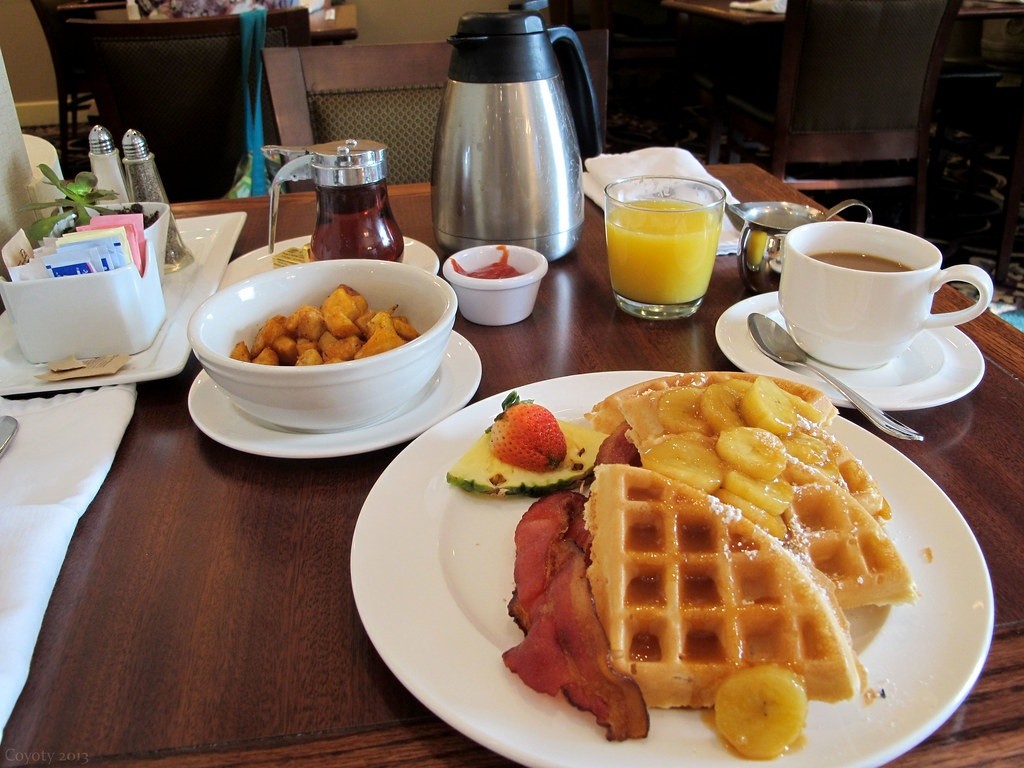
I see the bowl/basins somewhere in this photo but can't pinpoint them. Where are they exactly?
[186,257,458,429]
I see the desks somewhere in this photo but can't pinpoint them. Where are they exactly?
[662,0,1024,188]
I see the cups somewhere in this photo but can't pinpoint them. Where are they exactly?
[442,244,548,327]
[603,176,727,321]
[779,220,994,371]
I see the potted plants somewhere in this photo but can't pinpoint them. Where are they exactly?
[15,161,170,286]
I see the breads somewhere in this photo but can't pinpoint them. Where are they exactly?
[583,370,917,708]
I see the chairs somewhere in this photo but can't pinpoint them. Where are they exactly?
[29,0,1023,291]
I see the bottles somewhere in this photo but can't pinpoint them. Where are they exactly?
[88,125,193,274]
[261,138,404,261]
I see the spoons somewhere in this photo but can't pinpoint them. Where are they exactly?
[747,313,925,443]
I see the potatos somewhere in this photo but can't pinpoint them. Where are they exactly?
[230,284,420,366]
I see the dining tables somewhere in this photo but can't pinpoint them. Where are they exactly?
[0,163,1024,768]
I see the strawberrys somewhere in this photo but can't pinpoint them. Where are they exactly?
[489,401,566,472]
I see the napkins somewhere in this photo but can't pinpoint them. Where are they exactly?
[582,146,746,255]
[0,382,138,746]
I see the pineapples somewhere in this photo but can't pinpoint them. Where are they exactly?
[446,417,612,497]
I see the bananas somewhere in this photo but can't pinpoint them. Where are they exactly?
[710,666,805,760]
[639,375,829,544]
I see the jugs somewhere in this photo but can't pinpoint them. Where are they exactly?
[430,9,603,263]
[727,199,873,296]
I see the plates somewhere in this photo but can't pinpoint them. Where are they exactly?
[0,211,247,396]
[188,329,482,459]
[350,370,995,768]
[714,291,986,412]
[217,235,440,276]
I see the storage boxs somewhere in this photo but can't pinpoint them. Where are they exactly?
[0,238,167,364]
